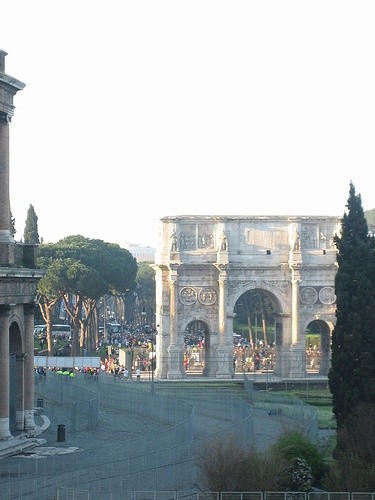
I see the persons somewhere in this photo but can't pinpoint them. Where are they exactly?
[34,296,322,384]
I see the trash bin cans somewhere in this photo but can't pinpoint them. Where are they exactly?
[37,397,43,407]
[56,423,66,442]
